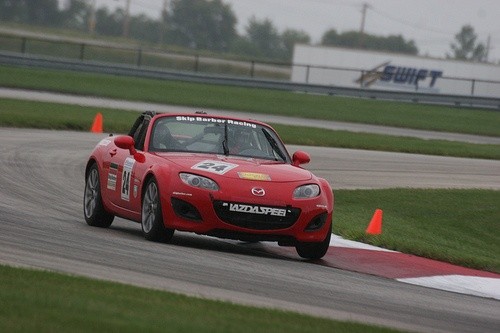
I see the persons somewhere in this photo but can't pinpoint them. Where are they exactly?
[228,129,253,154]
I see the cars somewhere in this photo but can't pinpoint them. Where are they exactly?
[83,110,334,259]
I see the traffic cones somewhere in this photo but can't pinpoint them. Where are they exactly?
[365,209,382,235]
[90,113,102,133]
